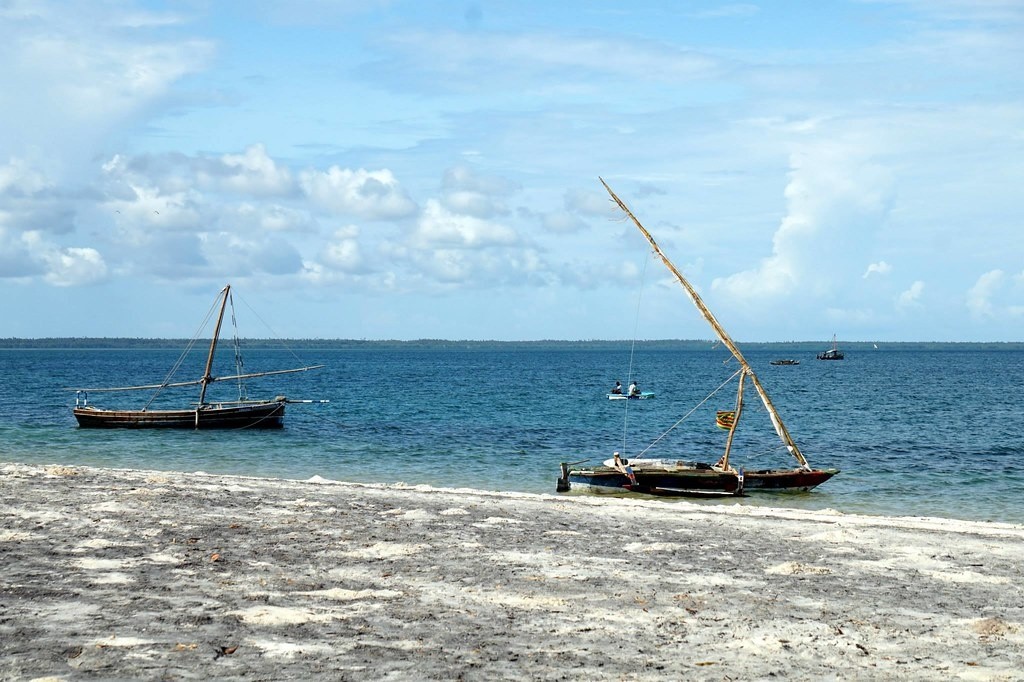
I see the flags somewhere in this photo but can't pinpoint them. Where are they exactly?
[717,409,735,429]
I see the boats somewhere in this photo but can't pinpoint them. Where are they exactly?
[769,358,800,365]
[607,392,656,400]
[563,171,840,498]
[816,333,845,360]
[61,282,331,430]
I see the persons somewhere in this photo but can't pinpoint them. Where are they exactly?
[630,381,639,396]
[612,381,623,393]
